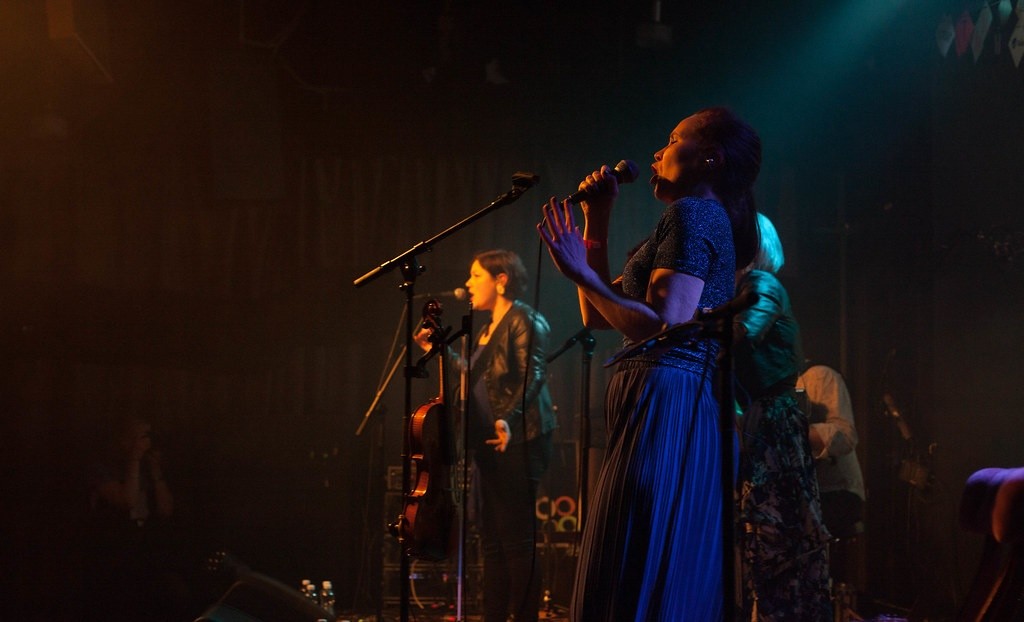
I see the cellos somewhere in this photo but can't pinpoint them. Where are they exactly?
[399,295,454,556]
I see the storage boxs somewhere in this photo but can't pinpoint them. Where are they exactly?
[382,467,482,614]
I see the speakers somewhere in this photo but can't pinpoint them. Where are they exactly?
[192,574,336,622]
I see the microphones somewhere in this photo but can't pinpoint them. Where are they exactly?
[612,291,761,361]
[414,288,467,301]
[883,391,913,442]
[570,158,641,205]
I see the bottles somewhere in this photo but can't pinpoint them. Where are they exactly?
[305,584,318,605]
[300,580,311,593]
[320,581,336,615]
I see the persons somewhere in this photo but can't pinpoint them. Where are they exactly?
[799,354,866,533]
[413,250,557,622]
[85,419,194,606]
[717,211,836,622]
[536,108,762,622]
